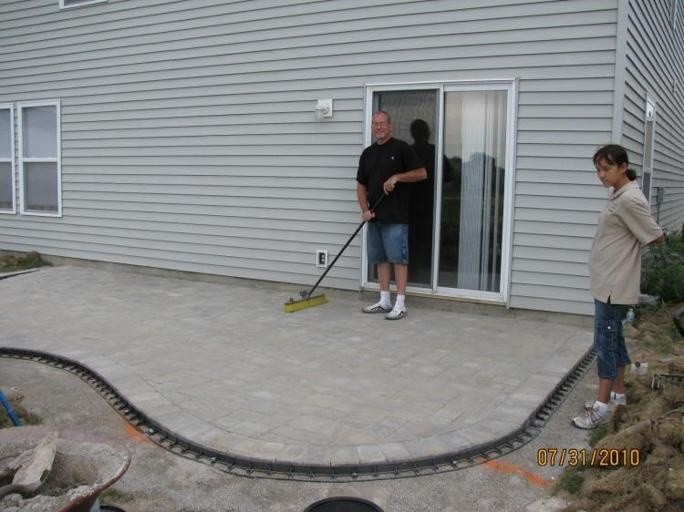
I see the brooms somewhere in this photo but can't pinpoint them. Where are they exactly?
[281,192,385,313]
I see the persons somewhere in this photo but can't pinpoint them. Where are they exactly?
[354,110,428,319]
[569,144,665,427]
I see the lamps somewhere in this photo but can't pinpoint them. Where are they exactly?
[314,99,333,118]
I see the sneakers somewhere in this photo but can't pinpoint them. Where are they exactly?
[361,303,392,313]
[583,394,627,411]
[385,307,408,321]
[571,407,612,429]
